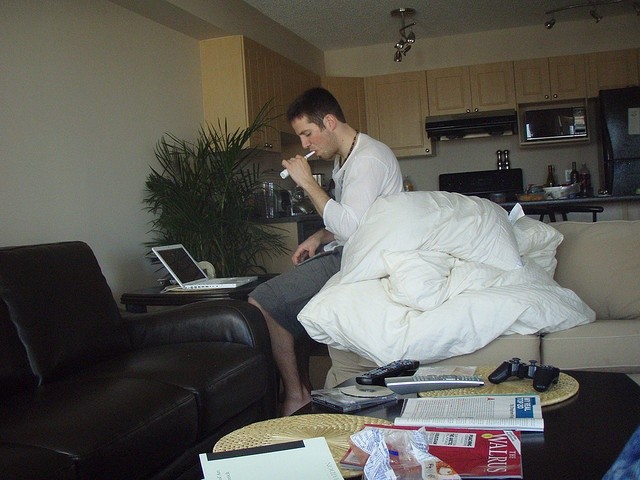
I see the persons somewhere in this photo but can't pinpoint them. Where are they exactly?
[246,87,403,419]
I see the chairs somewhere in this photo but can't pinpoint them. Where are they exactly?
[503,203,603,221]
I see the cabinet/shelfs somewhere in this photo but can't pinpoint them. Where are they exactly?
[249,212,325,357]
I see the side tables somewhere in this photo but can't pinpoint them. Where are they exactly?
[122,273,279,314]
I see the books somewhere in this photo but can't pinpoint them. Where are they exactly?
[393,393,545,431]
[339,422,525,479]
[198,435,344,480]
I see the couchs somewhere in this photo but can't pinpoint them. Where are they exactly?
[323,221,639,367]
[1,241,276,479]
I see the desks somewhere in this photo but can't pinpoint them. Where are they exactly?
[287,370,640,479]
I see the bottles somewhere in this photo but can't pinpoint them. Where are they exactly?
[570,162,580,185]
[580,161,591,197]
[403,174,413,191]
[546,166,556,188]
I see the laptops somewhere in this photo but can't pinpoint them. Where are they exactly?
[151,243,259,289]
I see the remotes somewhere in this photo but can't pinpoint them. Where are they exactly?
[384,375,485,396]
[356,358,420,386]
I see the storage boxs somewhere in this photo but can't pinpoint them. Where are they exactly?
[515,51,585,104]
[323,69,434,158]
[426,61,517,118]
[199,35,323,153]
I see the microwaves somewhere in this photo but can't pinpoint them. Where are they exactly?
[524,107,588,142]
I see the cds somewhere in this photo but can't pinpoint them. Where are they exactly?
[340,386,394,398]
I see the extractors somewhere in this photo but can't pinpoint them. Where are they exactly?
[424,109,519,140]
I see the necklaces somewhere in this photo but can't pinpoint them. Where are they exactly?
[339,130,359,169]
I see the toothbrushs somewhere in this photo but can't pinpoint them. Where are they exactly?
[278,150,317,180]
